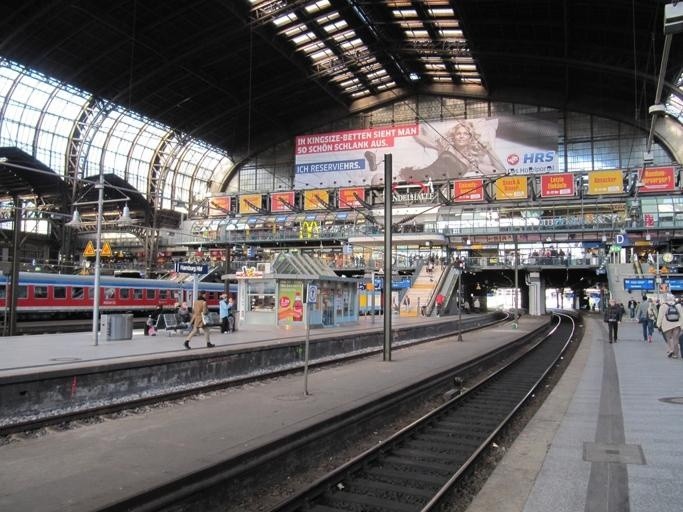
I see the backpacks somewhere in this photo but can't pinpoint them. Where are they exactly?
[647,303,658,321]
[663,302,680,322]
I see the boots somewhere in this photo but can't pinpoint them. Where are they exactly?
[207,342,215,348]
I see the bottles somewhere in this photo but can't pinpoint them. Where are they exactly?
[292,291,303,323]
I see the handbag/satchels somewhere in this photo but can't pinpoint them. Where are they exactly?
[201,312,219,325]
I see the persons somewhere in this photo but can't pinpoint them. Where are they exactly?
[461,298,481,314]
[403,294,410,312]
[183,290,215,349]
[396,120,506,181]
[604,292,683,358]
[326,246,571,276]
[219,294,233,334]
[435,293,444,316]
[177,301,199,335]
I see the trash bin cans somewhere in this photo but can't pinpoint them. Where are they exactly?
[110,314,134,341]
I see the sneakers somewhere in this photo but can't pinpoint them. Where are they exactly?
[667,351,678,359]
[183,340,191,349]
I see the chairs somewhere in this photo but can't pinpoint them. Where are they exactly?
[152,311,231,335]
